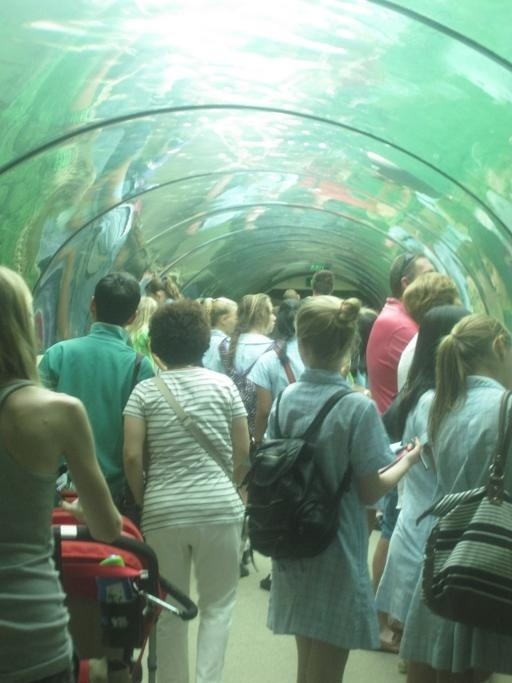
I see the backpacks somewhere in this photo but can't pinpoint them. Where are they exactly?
[237,387,362,562]
[216,336,276,440]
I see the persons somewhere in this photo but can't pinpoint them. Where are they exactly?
[1,253,512,682]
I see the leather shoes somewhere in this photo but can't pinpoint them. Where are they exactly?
[374,626,404,655]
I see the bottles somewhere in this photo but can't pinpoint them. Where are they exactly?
[97,553,139,625]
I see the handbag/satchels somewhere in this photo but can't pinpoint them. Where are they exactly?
[413,389,512,633]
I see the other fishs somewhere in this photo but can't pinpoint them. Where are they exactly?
[298,173,379,218]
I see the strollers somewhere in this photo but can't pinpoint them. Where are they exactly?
[52,503,199,682]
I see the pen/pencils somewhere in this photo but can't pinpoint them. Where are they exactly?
[411,438,429,470]
